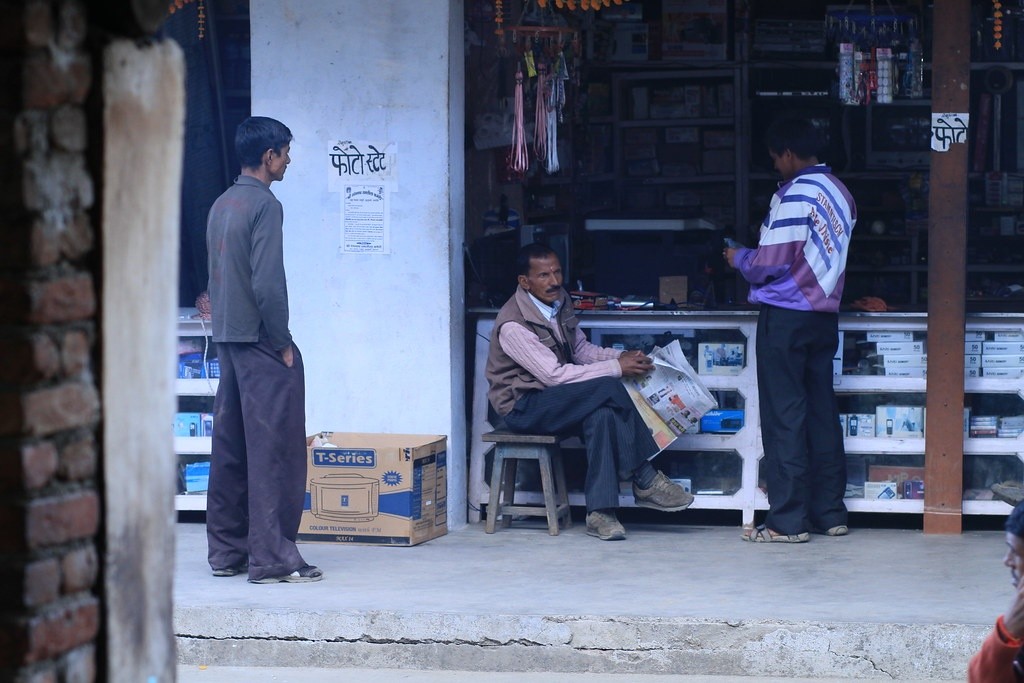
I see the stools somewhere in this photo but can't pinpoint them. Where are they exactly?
[483,432,573,536]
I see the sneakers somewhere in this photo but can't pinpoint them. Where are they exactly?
[585,511,627,540]
[632,470,694,513]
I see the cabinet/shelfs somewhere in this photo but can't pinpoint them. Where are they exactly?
[468,305,1024,544]
[742,0,1024,305]
[571,0,742,221]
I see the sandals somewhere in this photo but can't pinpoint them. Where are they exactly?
[823,525,848,536]
[739,524,809,542]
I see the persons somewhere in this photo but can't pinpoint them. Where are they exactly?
[681,411,697,424]
[704,345,739,371]
[841,296,887,311]
[486,244,694,540]
[206,116,323,584]
[968,501,1024,683]
[724,119,857,542]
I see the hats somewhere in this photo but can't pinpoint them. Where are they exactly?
[1004,498,1024,539]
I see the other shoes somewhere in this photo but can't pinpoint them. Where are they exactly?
[247,563,323,584]
[211,555,248,576]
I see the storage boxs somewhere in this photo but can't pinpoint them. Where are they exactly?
[984,172,1024,206]
[185,460,210,492]
[625,84,734,119]
[295,432,446,547]
[698,341,744,375]
[179,338,205,379]
[622,128,734,177]
[620,478,692,496]
[173,305,219,524]
[688,410,744,435]
[867,333,1024,381]
[622,186,735,220]
[839,404,1024,441]
[844,459,924,499]
[175,412,213,437]
[585,0,731,61]
